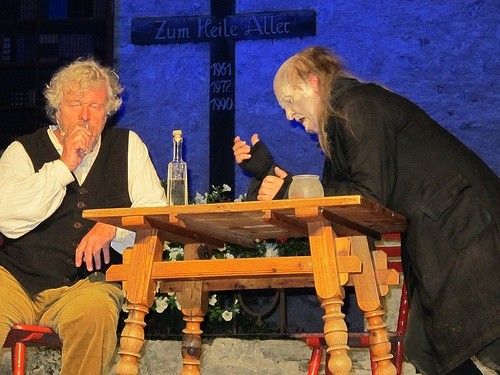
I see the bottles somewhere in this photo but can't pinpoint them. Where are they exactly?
[166,130,188,206]
[288,174,324,199]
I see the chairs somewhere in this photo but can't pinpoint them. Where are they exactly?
[3,322,63,374]
[305,232,409,375]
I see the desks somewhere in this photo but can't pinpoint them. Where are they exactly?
[82,195,409,375]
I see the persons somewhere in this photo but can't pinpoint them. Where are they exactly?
[1,59,168,375]
[234,46,500,375]
